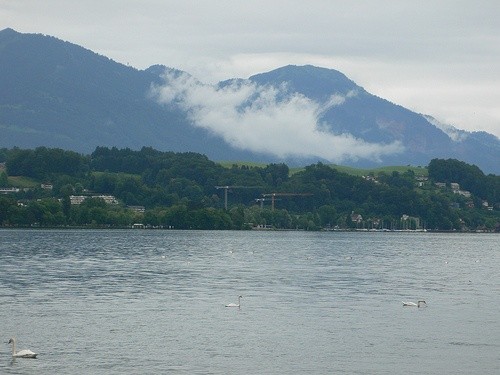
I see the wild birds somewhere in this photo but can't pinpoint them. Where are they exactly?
[8,337,39,358]
[402,300,426,307]
[225,296,242,307]
